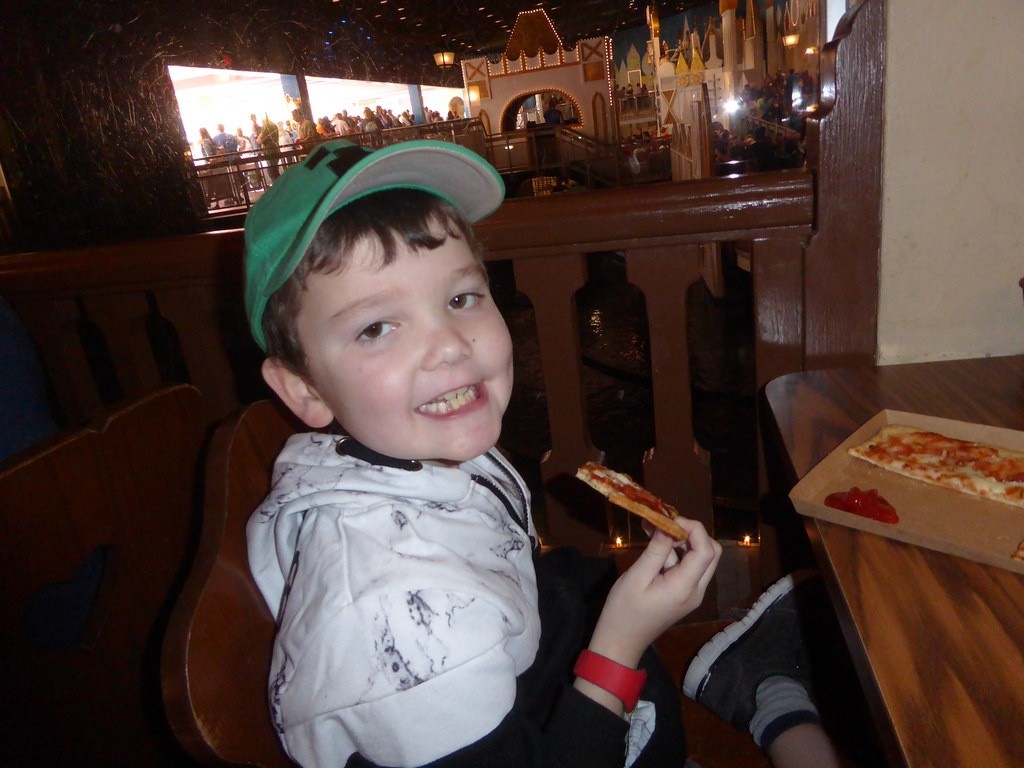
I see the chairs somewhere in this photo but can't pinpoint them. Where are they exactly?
[162,395,758,768]
[0,386,215,768]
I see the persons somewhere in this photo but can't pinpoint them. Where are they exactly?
[618,126,673,163]
[683,566,854,768]
[197,105,469,210]
[237,135,723,768]
[544,103,564,125]
[707,66,821,173]
[614,80,652,114]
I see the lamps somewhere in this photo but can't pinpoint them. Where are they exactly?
[782,31,800,50]
[432,37,456,70]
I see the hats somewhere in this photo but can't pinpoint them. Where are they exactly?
[244,137,505,352]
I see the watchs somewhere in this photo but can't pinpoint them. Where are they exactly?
[573,648,647,715]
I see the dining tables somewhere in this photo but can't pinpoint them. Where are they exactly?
[763,354,1024,768]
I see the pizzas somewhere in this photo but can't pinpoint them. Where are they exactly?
[848,424,1024,508]
[575,461,689,540]
